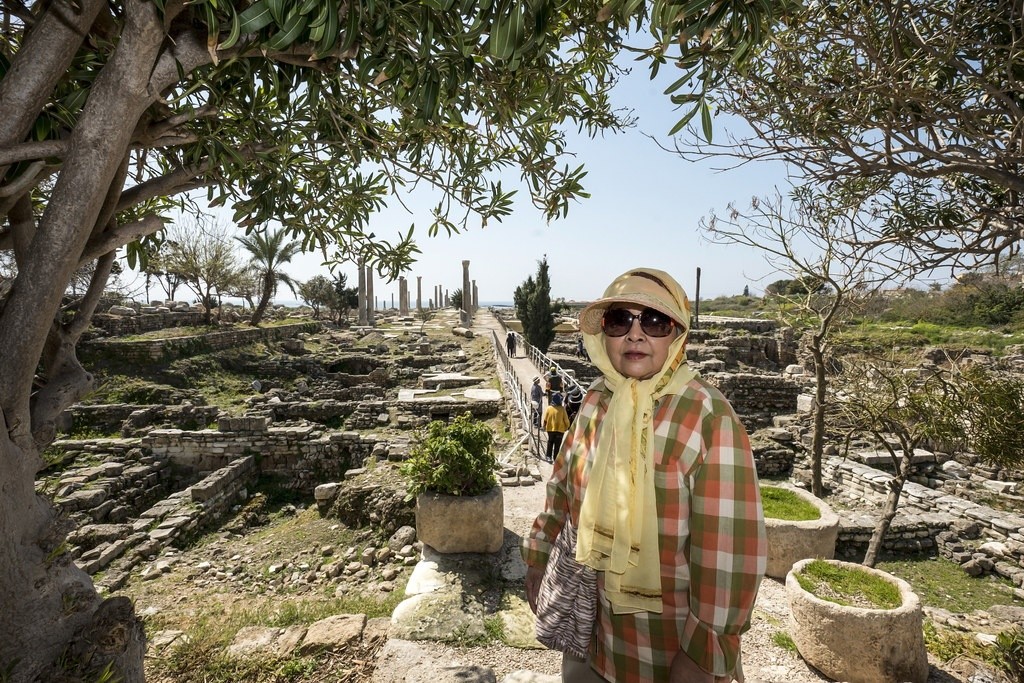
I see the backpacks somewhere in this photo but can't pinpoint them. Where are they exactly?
[568,402,581,426]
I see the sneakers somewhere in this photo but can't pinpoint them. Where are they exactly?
[546,457,552,461]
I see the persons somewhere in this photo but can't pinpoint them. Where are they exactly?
[505,333,517,358]
[544,395,570,463]
[531,367,583,430]
[524,266,766,683]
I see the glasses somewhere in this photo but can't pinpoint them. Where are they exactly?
[602,307,678,338]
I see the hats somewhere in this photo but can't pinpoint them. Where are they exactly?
[551,367,555,371]
[532,376,540,382]
[580,275,687,335]
[568,390,584,403]
[551,392,563,406]
[567,385,579,394]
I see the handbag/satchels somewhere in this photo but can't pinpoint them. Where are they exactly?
[535,514,597,660]
[531,400,539,409]
